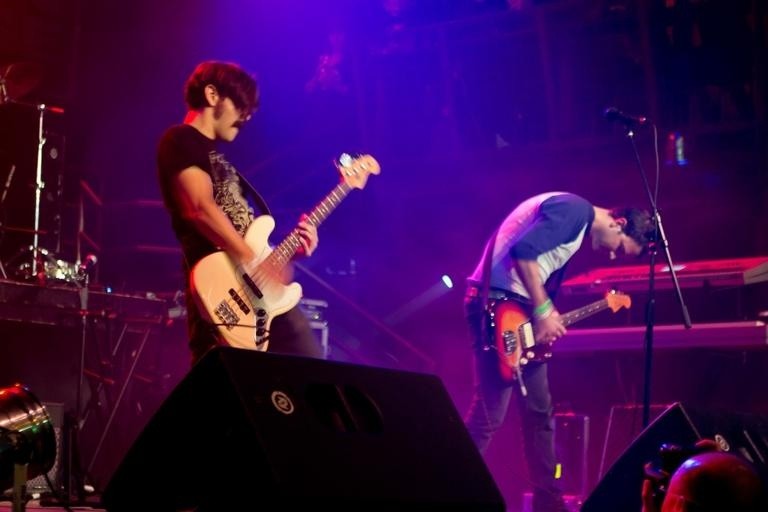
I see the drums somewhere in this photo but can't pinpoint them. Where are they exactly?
[0,383,56,492]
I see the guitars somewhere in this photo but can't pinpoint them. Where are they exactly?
[487,287,631,386]
[189,152,382,353]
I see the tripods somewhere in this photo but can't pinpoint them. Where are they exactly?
[0,98,85,290]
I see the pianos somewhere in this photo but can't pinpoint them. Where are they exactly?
[548,258,768,356]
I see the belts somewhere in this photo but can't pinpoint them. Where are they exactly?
[464,284,507,300]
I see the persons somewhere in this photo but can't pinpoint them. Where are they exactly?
[461,192,655,512]
[151,57,327,365]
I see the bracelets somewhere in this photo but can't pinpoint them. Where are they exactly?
[534,304,556,322]
[531,297,552,315]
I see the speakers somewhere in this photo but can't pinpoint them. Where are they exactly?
[579,400,768,512]
[100,345,506,512]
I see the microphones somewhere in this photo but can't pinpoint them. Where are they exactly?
[78,254,97,277]
[605,107,648,125]
